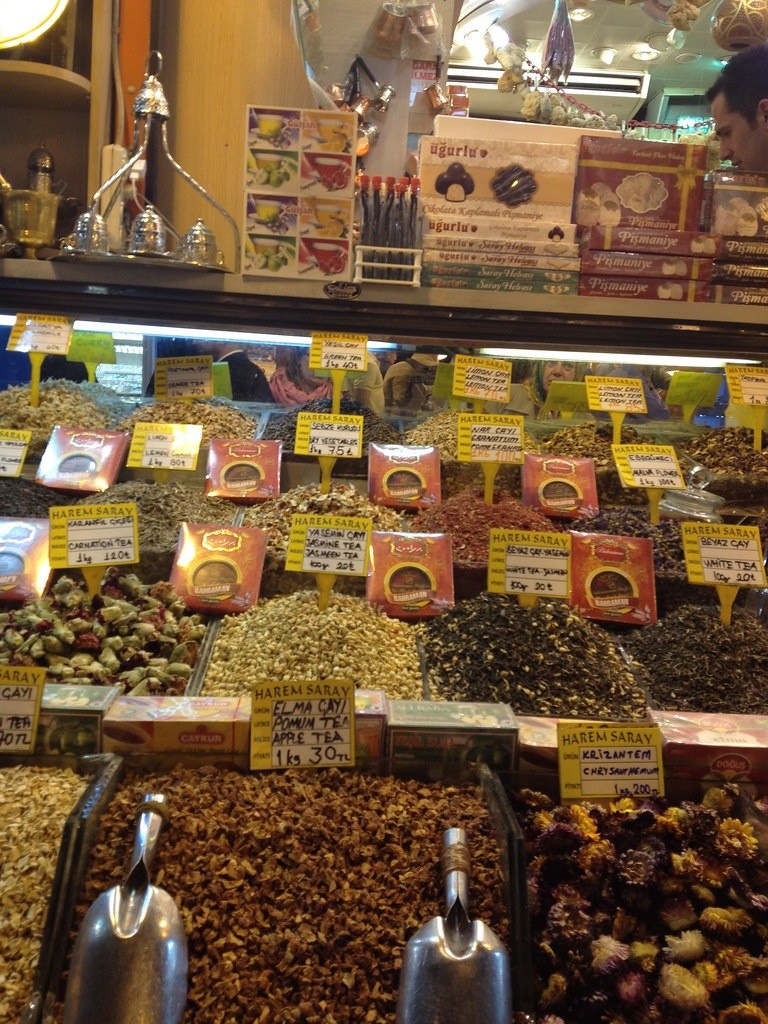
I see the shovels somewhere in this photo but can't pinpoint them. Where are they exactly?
[398,825,510,1024]
[64,792,190,1023]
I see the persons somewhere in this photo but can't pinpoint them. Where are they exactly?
[708,44,768,175]
[0,325,670,421]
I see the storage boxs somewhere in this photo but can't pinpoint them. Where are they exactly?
[413,113,768,306]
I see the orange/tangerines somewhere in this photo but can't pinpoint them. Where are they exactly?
[307,208,347,237]
[315,123,354,153]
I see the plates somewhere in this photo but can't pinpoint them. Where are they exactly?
[247,168,287,174]
[248,213,282,224]
[310,131,351,143]
[250,128,291,140]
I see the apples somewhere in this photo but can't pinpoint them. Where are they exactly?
[251,252,284,271]
[254,162,286,187]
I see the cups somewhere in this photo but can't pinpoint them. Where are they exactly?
[315,204,339,225]
[254,238,287,258]
[312,242,345,263]
[74,202,225,269]
[256,154,281,174]
[317,119,344,139]
[255,199,280,219]
[257,114,283,136]
[315,157,352,191]
